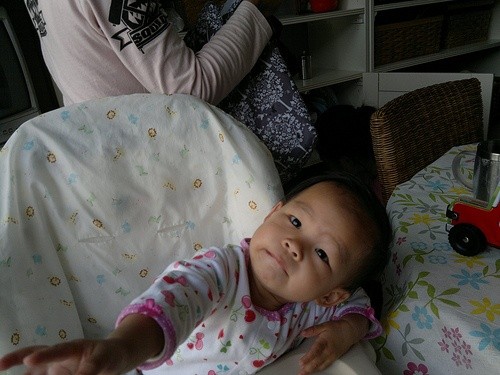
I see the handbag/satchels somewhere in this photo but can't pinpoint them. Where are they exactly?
[185,3,318,167]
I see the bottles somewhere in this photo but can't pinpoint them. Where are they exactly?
[299,50,311,80]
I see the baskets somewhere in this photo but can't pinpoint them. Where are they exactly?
[375,2,493,66]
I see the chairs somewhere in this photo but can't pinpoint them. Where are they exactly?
[371,78,486,208]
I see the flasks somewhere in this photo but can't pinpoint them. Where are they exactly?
[472,140,500,203]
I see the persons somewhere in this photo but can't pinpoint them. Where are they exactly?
[1,173,395,375]
[24,0,275,107]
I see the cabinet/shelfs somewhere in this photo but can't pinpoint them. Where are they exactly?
[265,0,500,170]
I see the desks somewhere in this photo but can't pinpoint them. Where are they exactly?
[392,143,500,375]
